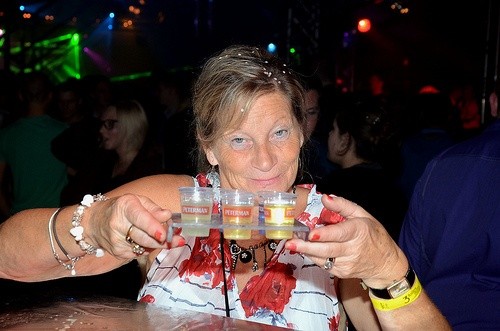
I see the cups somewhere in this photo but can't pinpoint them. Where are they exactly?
[220,192,255,240]
[256,190,278,235]
[262,192,298,240]
[216,188,244,232]
[179,186,215,237]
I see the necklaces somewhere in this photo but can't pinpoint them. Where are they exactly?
[229,184,297,271]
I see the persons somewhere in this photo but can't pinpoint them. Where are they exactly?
[0,45,455,331]
[0,55,197,313]
[398,80,500,331]
[297,69,473,248]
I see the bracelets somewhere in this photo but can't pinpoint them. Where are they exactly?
[48,206,84,276]
[368,271,422,310]
[70,192,110,258]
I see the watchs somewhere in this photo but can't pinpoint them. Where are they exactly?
[359,265,415,299]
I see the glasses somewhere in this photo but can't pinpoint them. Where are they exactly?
[93,119,117,129]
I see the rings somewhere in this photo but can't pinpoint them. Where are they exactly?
[322,258,335,270]
[125,225,145,254]
